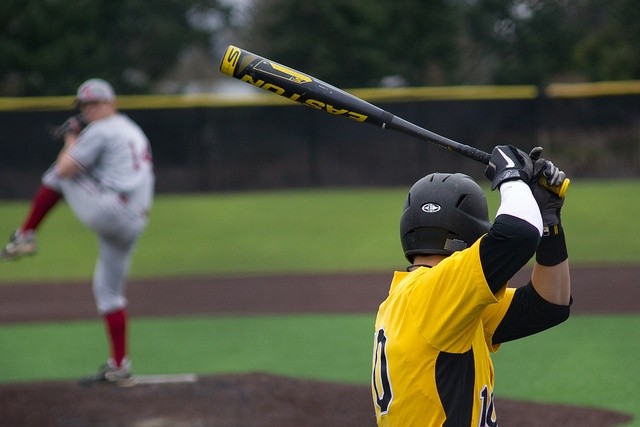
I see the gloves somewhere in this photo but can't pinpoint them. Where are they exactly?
[485,144,533,190]
[526,147,566,238]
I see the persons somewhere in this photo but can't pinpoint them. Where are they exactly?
[370,145,572,426]
[4,77,156,380]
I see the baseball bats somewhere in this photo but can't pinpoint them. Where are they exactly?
[218,41,570,199]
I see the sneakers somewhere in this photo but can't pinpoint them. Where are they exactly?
[78,362,137,390]
[5,234,36,256]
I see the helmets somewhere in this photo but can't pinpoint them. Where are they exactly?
[75,78,117,104]
[400,172,490,264]
